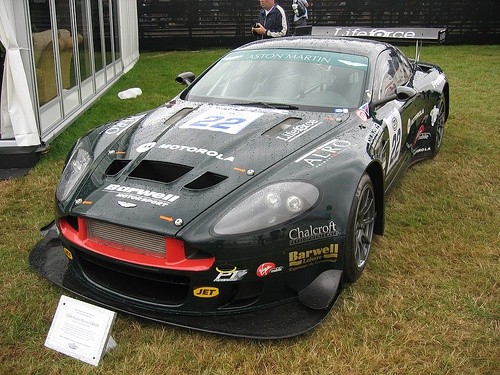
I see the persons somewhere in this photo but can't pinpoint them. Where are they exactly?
[251,0,289,41]
[292,0,309,28]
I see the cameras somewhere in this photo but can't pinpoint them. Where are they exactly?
[250,20,260,28]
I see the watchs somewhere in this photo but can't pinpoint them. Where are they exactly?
[264,29,266,35]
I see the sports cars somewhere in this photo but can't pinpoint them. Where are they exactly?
[28,26,450,341]
